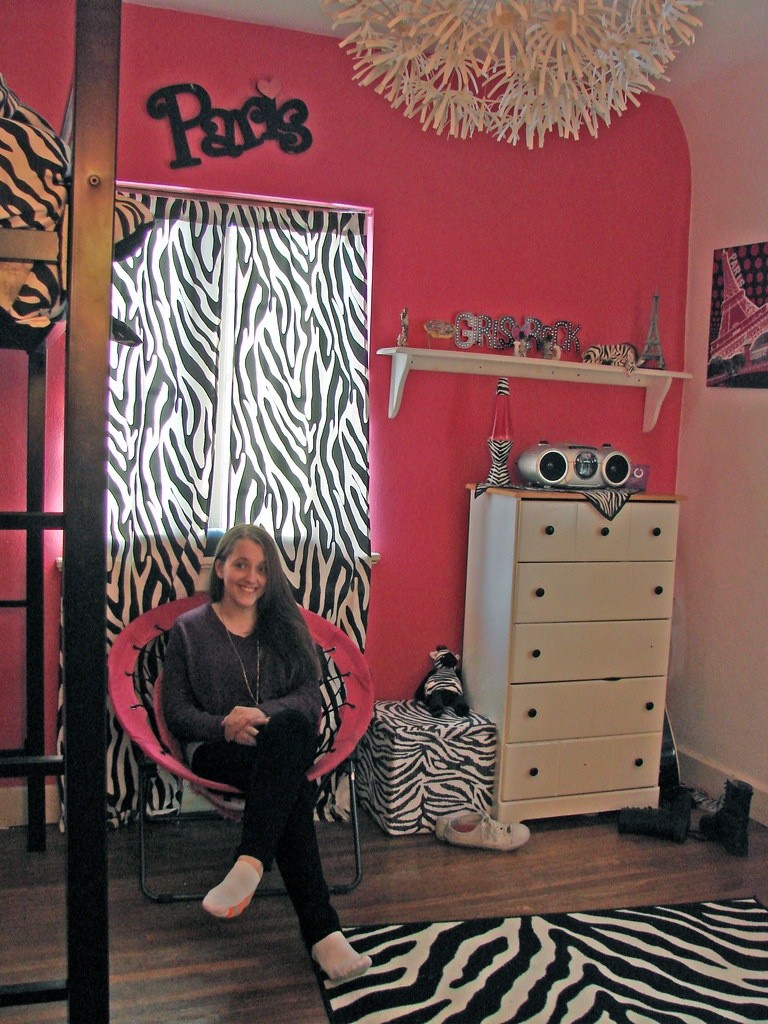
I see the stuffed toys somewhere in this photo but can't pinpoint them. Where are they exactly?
[582,342,638,375]
[413,645,471,718]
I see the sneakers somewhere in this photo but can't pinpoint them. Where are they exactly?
[436,809,478,842]
[446,808,531,850]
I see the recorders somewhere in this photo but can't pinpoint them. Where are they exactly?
[519,441,631,490]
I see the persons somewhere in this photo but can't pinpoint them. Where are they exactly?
[397,308,409,346]
[161,524,372,981]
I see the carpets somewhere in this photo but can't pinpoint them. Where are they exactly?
[314,894,768,1024]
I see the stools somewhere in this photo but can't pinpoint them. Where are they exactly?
[354,699,496,837]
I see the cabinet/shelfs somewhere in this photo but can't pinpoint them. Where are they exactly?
[461,483,688,824]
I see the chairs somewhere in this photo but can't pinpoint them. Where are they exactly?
[104,592,374,904]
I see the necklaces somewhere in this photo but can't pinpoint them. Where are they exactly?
[218,604,262,708]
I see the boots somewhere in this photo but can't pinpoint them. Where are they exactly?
[698,779,754,856]
[618,789,692,843]
